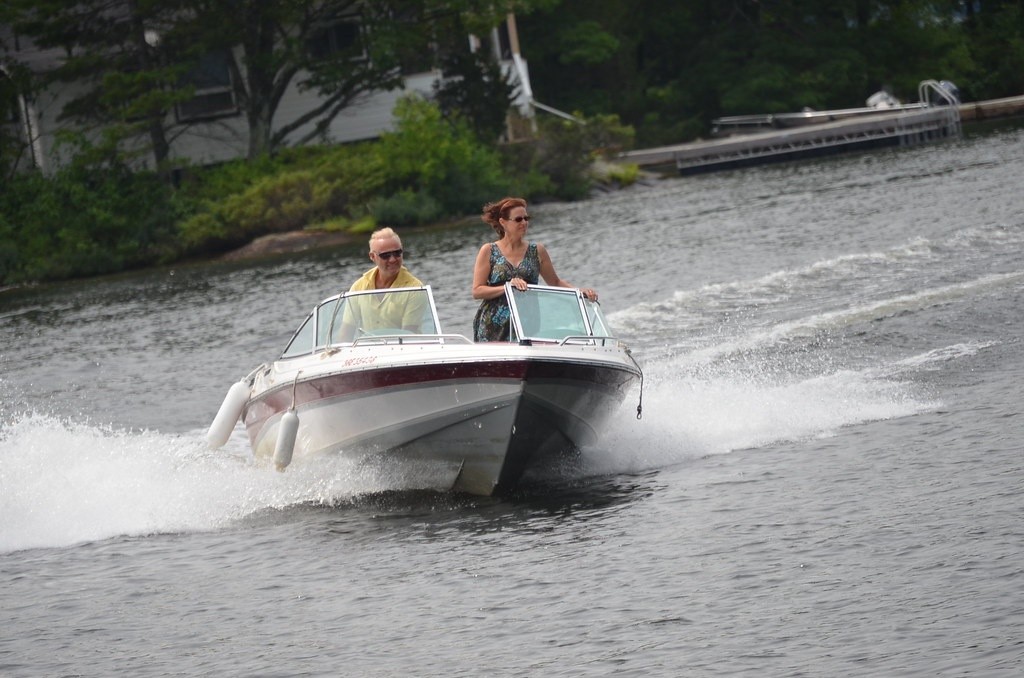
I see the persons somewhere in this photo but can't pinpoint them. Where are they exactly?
[472,198,598,343]
[337,227,426,343]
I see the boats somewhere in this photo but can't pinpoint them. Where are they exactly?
[203,284,644,512]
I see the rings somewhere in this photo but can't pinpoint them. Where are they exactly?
[590,291,592,292]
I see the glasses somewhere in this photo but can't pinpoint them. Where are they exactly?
[372,249,403,260]
[503,216,531,223]
